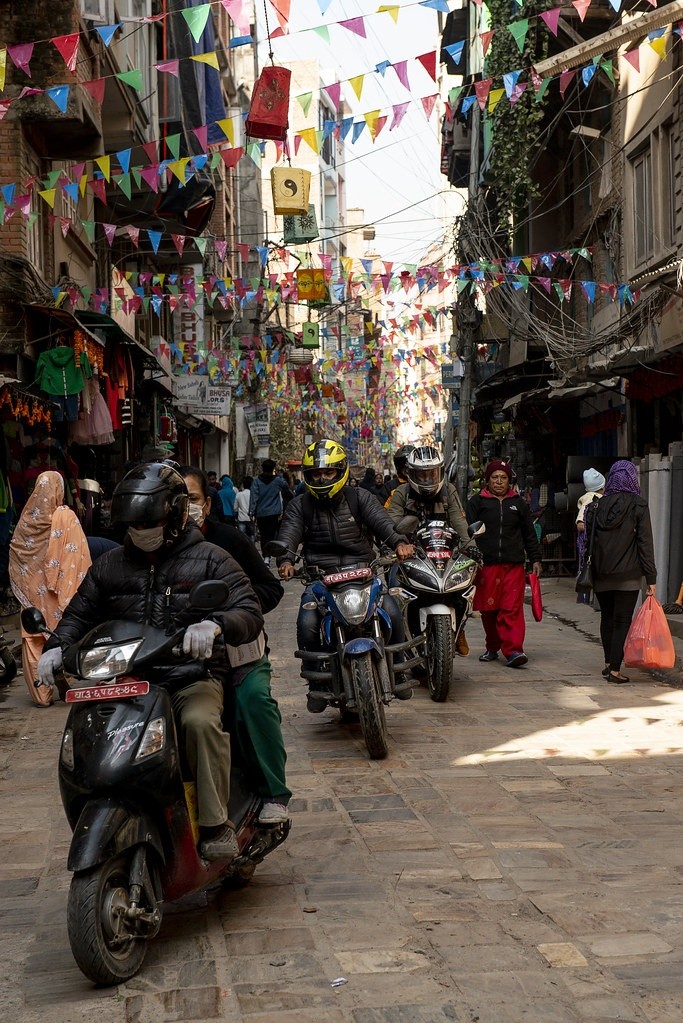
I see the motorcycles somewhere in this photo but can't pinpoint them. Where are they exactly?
[374,521,487,703]
[266,517,418,762]
[21,581,292,988]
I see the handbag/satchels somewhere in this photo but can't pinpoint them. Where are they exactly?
[623,595,675,669]
[575,564,593,593]
[528,574,543,622]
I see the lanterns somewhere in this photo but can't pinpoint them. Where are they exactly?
[361,427,373,437]
[322,383,347,403]
[289,322,319,366]
[295,369,310,383]
[337,415,347,423]
[245,66,292,140]
[297,266,326,300]
[307,287,332,309]
[271,166,320,246]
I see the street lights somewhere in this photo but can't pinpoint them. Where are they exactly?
[336,307,372,412]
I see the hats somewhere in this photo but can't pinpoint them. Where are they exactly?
[484,460,512,484]
[582,468,606,492]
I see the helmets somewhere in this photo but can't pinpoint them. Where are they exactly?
[301,439,350,500]
[405,446,446,498]
[111,463,189,556]
[393,445,418,481]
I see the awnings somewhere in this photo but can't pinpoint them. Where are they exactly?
[469,346,653,428]
[440,8,467,64]
[26,304,180,400]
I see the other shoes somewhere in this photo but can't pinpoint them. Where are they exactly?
[394,670,413,700]
[307,684,328,713]
[264,561,270,569]
[479,650,498,662]
[607,673,630,684]
[455,631,469,656]
[506,652,528,667]
[602,666,610,676]
[259,801,289,823]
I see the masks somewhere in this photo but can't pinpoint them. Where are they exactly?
[188,502,207,528]
[128,526,164,552]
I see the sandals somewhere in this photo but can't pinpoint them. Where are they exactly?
[199,819,239,859]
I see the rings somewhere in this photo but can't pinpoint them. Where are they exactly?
[282,573,284,575]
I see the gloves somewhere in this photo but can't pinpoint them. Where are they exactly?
[37,647,61,687]
[466,545,483,564]
[183,620,224,660]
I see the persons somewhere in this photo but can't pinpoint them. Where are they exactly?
[464,460,543,667]
[38,462,265,860]
[276,439,415,714]
[575,459,658,683]
[375,445,483,656]
[207,460,300,564]
[8,471,91,705]
[174,465,293,824]
[530,477,555,571]
[348,468,397,494]
